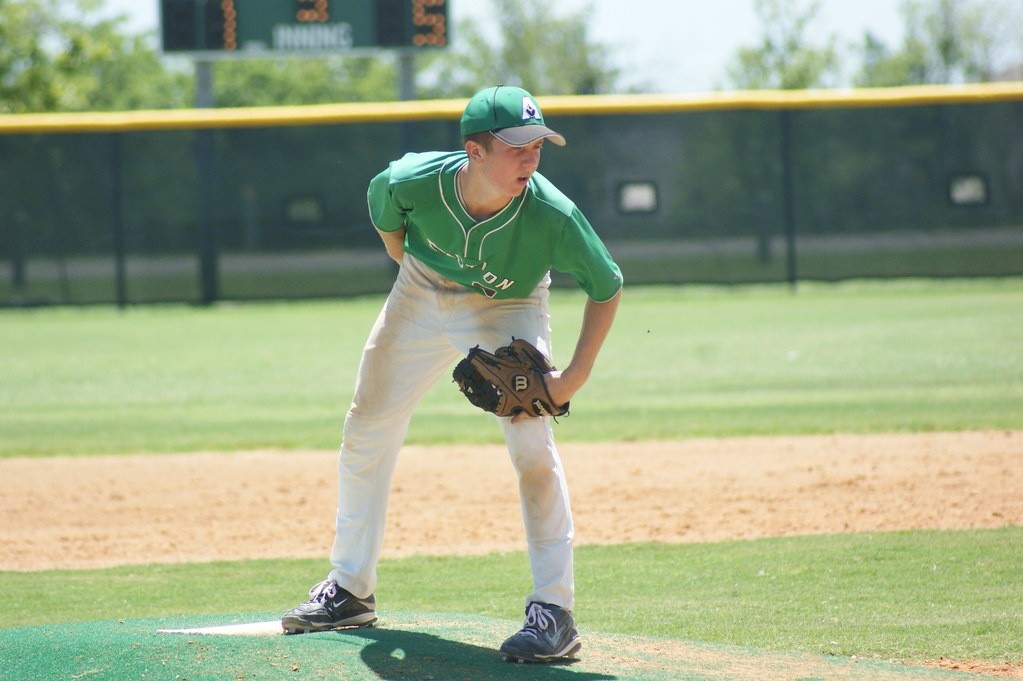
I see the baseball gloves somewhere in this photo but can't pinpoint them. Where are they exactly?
[452,336,570,424]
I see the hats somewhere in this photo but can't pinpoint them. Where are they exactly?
[459,84,567,147]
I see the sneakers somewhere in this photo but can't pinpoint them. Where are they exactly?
[499,601,581,662]
[281,579,378,630]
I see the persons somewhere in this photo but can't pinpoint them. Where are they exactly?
[281,84,623,661]
